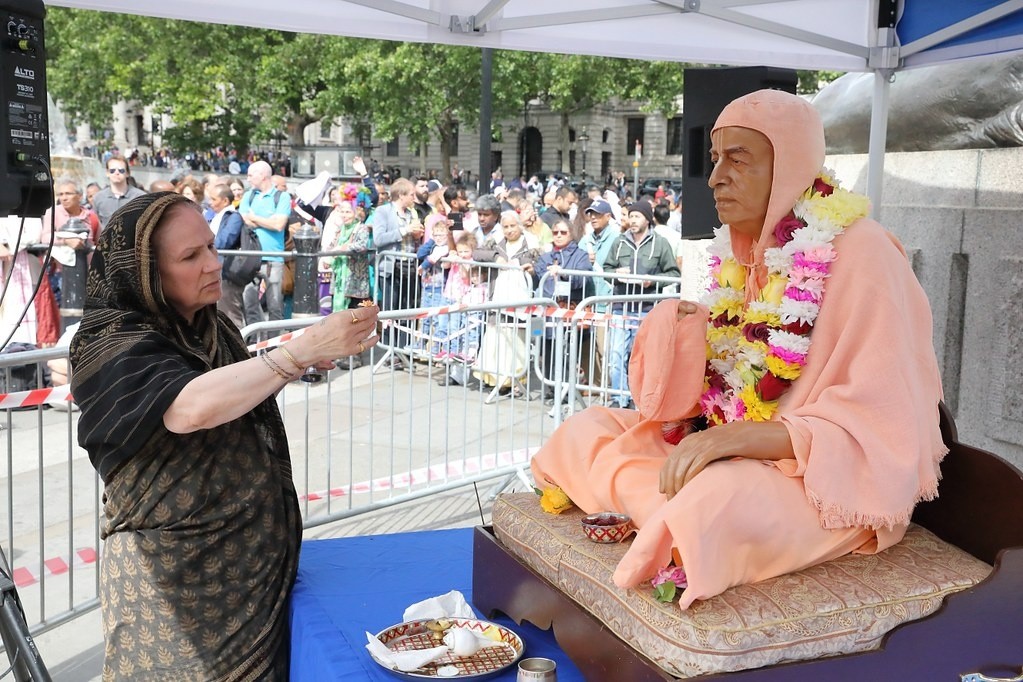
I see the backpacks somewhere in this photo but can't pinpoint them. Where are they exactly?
[222,212,262,286]
[249,189,290,243]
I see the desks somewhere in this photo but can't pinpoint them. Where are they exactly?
[278,521,586,682]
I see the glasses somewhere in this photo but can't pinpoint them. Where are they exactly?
[553,230,568,236]
[108,168,126,174]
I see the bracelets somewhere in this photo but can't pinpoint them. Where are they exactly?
[259,347,304,380]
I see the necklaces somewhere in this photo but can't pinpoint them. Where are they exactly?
[696,166,871,427]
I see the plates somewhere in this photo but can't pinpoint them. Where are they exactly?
[371,616,523,678]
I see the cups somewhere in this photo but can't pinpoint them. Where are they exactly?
[515,658,557,682]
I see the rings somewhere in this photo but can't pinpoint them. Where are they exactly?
[358,341,364,353]
[351,312,358,323]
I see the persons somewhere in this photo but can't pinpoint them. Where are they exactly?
[0,137,379,359]
[530,89,951,611]
[317,154,681,418]
[69,190,380,682]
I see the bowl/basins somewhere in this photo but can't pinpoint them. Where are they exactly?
[581,513,632,543]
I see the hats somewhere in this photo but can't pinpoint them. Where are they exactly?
[428,182,439,192]
[585,200,612,215]
[628,201,654,223]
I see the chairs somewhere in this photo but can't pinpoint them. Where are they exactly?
[473,399,1023,682]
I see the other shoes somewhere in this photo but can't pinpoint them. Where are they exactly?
[479,381,634,409]
[384,354,459,386]
